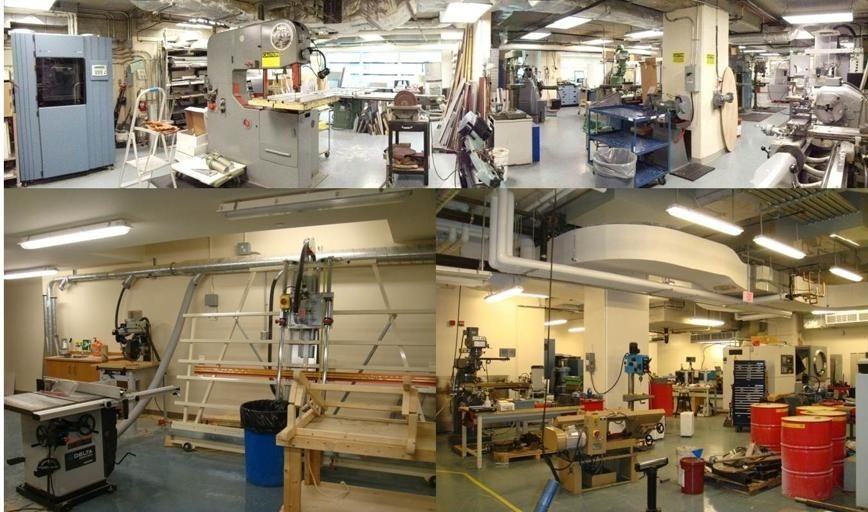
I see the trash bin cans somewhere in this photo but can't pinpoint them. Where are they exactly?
[240,400,299,487]
[593,148,636,188]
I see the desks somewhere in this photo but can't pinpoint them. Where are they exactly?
[387,113,430,185]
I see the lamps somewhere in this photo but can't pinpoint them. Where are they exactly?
[16,219,134,250]
[214,189,413,222]
[666,188,863,283]
[484,274,525,303]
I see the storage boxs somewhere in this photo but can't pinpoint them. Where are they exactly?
[174,106,207,163]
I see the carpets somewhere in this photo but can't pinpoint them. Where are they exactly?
[670,162,715,182]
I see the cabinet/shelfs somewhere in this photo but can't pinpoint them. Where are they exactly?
[161,47,207,124]
[558,84,582,105]
[47,360,100,382]
[588,105,671,187]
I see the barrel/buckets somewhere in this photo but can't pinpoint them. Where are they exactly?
[799,410,846,491]
[681,457,705,494]
[675,447,699,483]
[781,415,834,500]
[750,404,789,455]
